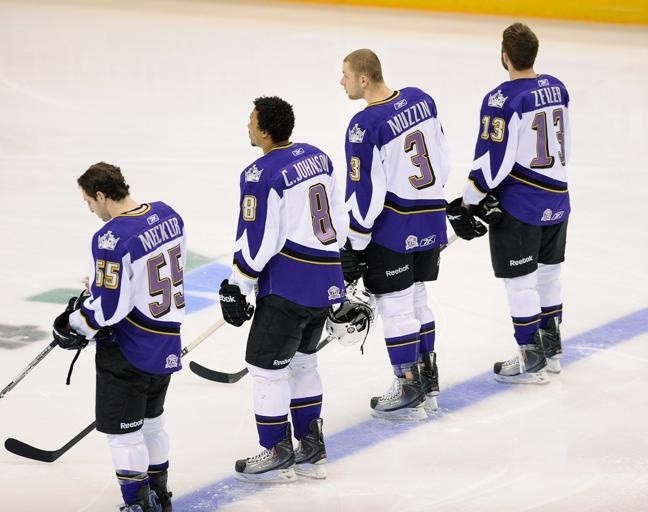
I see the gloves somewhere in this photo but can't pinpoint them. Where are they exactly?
[340,238,368,283]
[219,279,254,327]
[446,194,502,240]
[52,289,108,350]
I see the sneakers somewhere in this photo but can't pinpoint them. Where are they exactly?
[235,434,329,473]
[370,365,440,412]
[493,332,562,377]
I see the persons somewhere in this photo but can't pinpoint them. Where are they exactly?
[445,21,571,375]
[219,95,346,482]
[340,50,452,413]
[53,161,187,511]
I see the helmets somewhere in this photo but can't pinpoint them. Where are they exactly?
[326,284,375,342]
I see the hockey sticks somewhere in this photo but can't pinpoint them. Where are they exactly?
[190,262,367,384]
[4,303,254,462]
